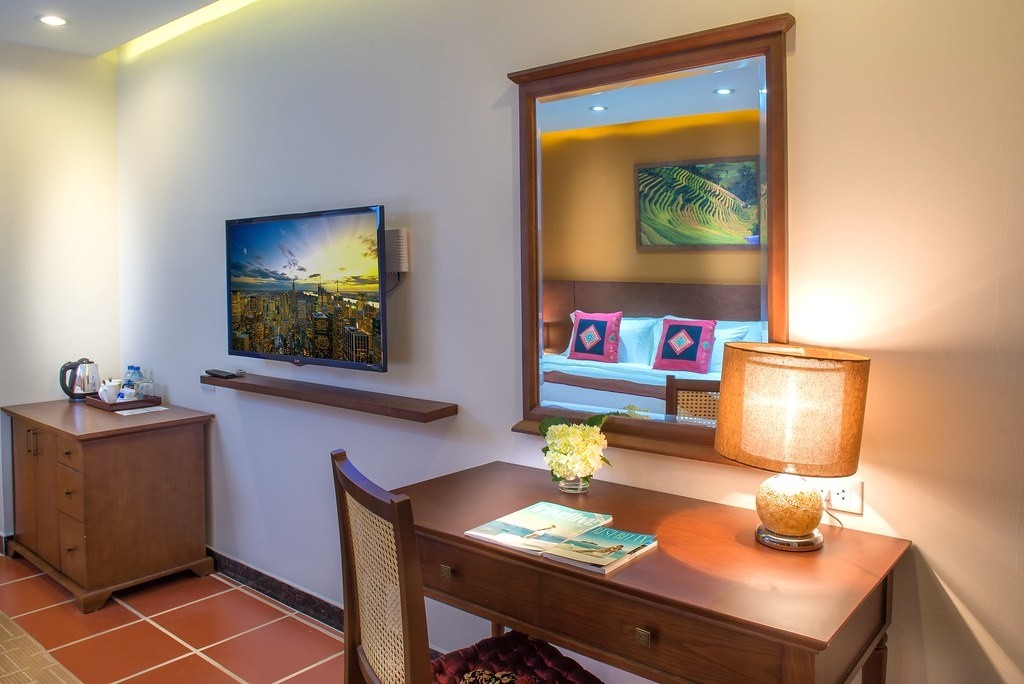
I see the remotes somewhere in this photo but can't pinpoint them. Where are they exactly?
[205,368,246,379]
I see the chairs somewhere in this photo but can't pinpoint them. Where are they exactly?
[330,448,606,684]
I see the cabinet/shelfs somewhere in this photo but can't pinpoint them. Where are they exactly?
[0,399,215,612]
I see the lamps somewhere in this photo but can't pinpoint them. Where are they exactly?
[712,342,871,552]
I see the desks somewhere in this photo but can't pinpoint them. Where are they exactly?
[384,460,913,684]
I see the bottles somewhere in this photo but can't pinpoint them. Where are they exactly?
[123,366,144,390]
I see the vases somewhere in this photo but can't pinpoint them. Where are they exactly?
[559,478,587,493]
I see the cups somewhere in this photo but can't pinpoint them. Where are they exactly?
[98,379,123,403]
[134,378,154,400]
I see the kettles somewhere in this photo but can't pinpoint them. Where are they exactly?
[59,358,100,402]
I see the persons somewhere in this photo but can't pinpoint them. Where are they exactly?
[521,525,556,539]
[573,545,624,555]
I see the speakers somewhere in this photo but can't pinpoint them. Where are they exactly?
[385,228,409,273]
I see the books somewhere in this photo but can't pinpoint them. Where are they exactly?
[543,526,658,575]
[464,501,614,558]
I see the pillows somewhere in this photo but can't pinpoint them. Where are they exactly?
[561,309,761,375]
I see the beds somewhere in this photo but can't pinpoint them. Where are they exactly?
[541,279,763,426]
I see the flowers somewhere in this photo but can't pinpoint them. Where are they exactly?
[539,412,614,486]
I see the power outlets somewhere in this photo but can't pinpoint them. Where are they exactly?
[819,481,864,514]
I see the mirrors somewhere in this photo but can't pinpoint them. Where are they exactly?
[507,13,797,469]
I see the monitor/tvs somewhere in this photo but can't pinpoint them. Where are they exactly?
[225,205,388,373]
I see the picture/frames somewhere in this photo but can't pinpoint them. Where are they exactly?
[633,155,762,251]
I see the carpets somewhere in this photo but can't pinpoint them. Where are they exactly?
[0,609,86,684]
